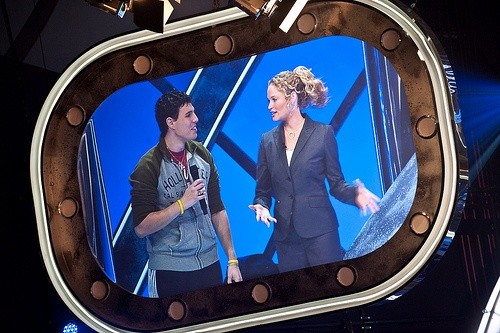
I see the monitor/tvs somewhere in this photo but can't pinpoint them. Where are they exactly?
[73,34,423,298]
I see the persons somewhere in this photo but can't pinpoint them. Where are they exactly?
[249,65,380,274]
[129,89,243,298]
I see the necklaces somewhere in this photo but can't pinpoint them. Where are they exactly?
[284,119,305,139]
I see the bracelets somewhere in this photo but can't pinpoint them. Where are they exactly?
[177,199,184,214]
[228,260,238,263]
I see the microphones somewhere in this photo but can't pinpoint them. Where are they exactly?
[190,165,208,215]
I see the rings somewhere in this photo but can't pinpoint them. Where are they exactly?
[198,190,201,195]
